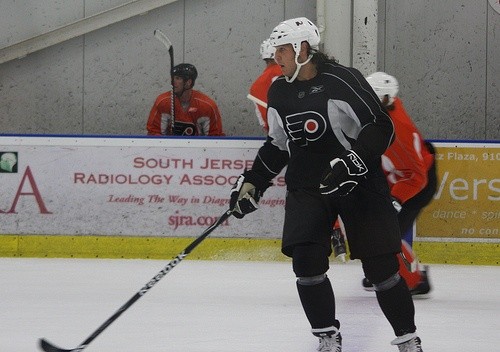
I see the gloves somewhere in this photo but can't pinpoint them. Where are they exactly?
[319,149,368,196]
[229,170,273,219]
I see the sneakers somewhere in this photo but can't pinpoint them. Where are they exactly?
[362,278,374,291]
[332,228,347,264]
[409,267,431,299]
[311,319,343,352]
[392,330,423,352]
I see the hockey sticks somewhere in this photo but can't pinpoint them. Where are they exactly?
[39,190,252,352]
[154,29,176,136]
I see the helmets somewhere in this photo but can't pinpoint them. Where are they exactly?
[270,17,320,53]
[173,63,198,81]
[260,38,277,59]
[366,72,398,105]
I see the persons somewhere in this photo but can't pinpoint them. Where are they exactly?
[228,17,423,352]
[364,71,438,294]
[248,39,348,262]
[146,63,229,136]
